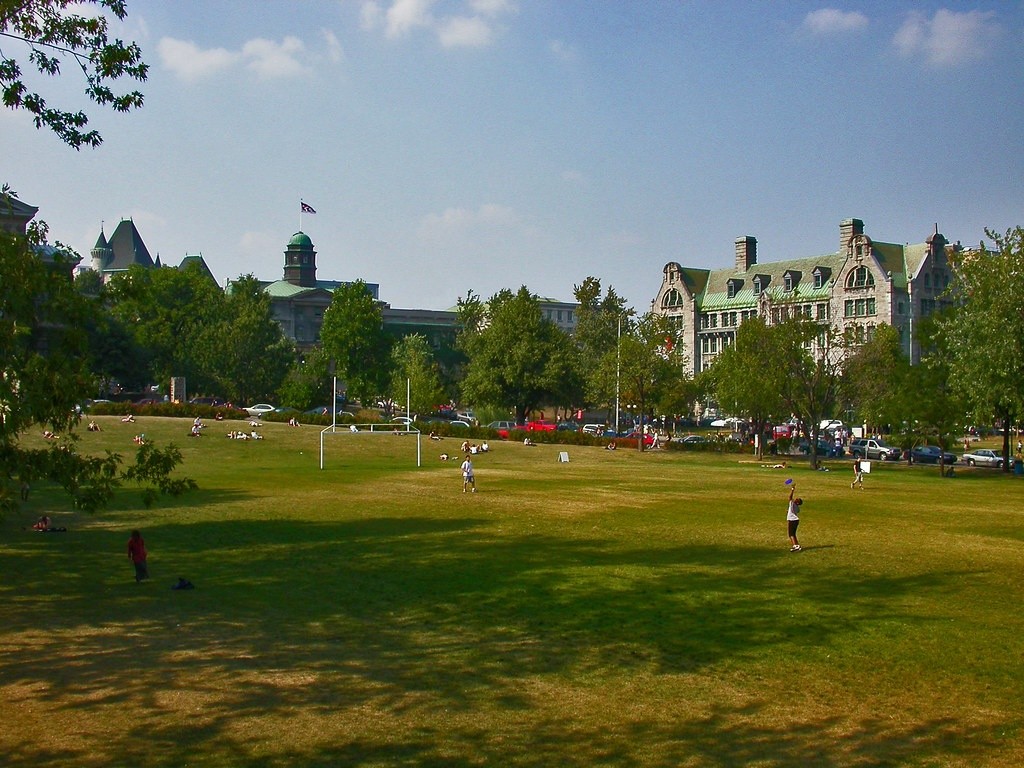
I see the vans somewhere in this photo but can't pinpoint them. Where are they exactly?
[817,420,843,431]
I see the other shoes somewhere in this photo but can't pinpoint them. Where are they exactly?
[463,489,466,492]
[790,544,802,552]
[851,483,854,489]
[472,487,478,492]
[859,486,863,489]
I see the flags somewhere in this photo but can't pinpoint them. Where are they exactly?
[301,203,317,214]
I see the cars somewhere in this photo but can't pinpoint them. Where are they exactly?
[582,424,613,437]
[848,439,902,461]
[903,446,957,465]
[239,404,294,419]
[457,412,476,422]
[526,420,558,433]
[798,439,845,458]
[962,449,1014,468]
[389,417,412,424]
[809,429,829,442]
[189,397,225,407]
[557,422,582,432]
[680,435,709,443]
[71,400,111,414]
[480,421,517,429]
[607,432,654,450]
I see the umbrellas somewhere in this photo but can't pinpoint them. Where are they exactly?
[712,418,744,426]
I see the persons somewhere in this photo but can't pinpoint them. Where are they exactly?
[767,428,881,471]
[122,415,134,422]
[90,421,101,431]
[45,431,60,439]
[459,455,476,492]
[414,411,659,460]
[1016,440,1023,458]
[135,436,145,444]
[787,484,802,552]
[128,530,147,583]
[38,515,52,531]
[851,455,864,489]
[192,398,399,440]
[965,436,969,450]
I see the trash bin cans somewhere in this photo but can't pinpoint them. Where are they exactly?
[1013,460,1023,475]
